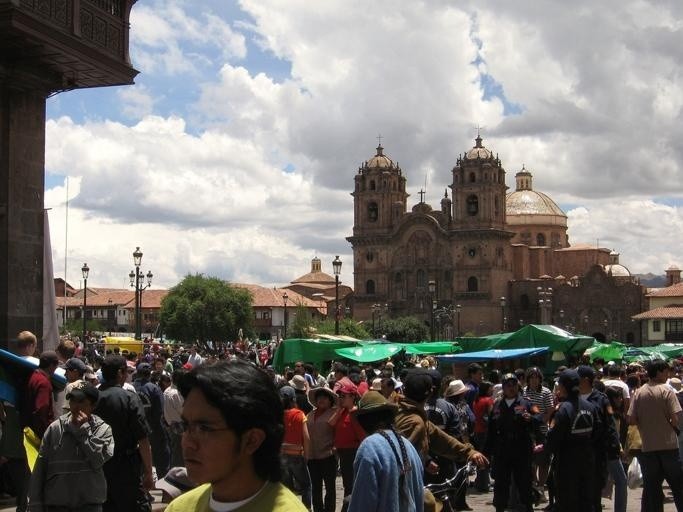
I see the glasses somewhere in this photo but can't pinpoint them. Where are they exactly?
[169,419,249,435]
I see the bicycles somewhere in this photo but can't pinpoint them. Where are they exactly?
[422,458,477,512]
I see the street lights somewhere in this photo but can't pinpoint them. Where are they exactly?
[107,297,114,336]
[81,261,90,349]
[332,255,343,335]
[455,303,462,337]
[344,305,351,319]
[134,244,143,340]
[368,303,375,337]
[282,291,288,339]
[374,303,390,325]
[432,299,437,340]
[79,302,82,319]
[494,285,611,338]
[127,269,153,339]
[426,278,434,343]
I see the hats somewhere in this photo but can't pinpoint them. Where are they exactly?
[38,350,63,369]
[335,382,358,396]
[57,357,88,374]
[501,372,520,386]
[279,385,296,400]
[308,386,338,410]
[100,355,131,372]
[153,466,201,501]
[65,380,100,404]
[665,377,683,394]
[442,379,472,399]
[557,364,596,385]
[286,374,308,392]
[137,362,152,370]
[347,389,400,420]
[401,367,437,401]
